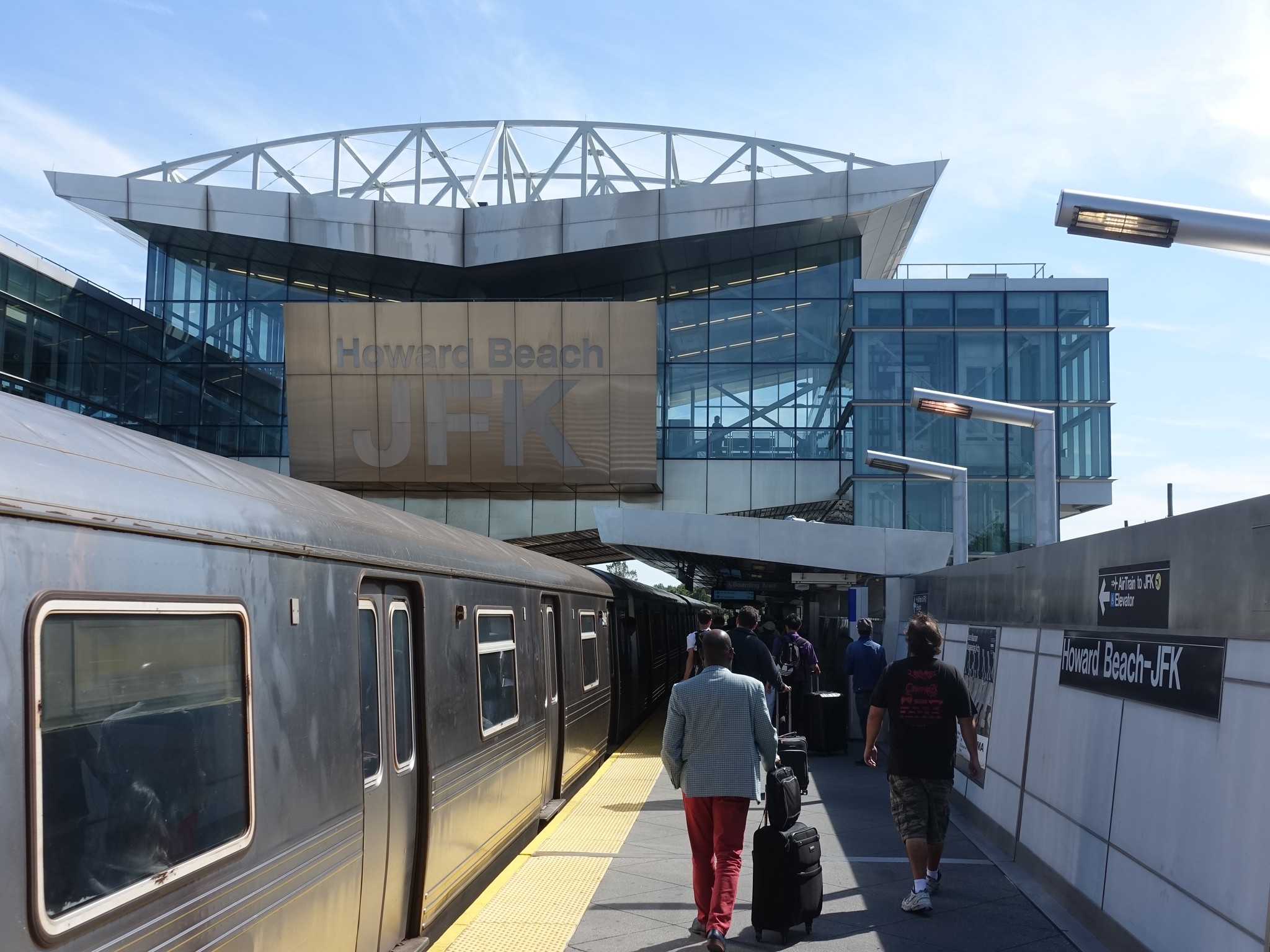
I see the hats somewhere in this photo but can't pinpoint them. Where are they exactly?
[856,618,873,631]
[713,616,725,625]
[764,621,776,630]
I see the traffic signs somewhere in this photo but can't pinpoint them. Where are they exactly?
[1096,559,1171,628]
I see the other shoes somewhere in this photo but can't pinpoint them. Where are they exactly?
[854,758,878,766]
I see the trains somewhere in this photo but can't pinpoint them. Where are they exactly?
[1,389,725,952]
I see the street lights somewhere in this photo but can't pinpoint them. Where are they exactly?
[864,450,969,567]
[910,386,1058,548]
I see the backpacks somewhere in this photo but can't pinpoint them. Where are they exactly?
[779,633,806,687]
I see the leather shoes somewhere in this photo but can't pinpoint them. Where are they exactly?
[692,917,708,938]
[706,928,727,952]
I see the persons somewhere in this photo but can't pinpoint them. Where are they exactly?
[662,629,777,951]
[843,618,887,767]
[766,614,821,736]
[713,612,787,654]
[725,606,791,694]
[678,608,714,683]
[863,611,981,911]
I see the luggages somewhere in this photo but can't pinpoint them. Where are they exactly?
[810,669,848,758]
[775,685,810,796]
[752,777,824,942]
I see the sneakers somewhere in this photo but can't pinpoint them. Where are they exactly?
[923,871,941,892]
[901,886,932,913]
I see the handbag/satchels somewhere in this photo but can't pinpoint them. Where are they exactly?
[766,763,801,829]
